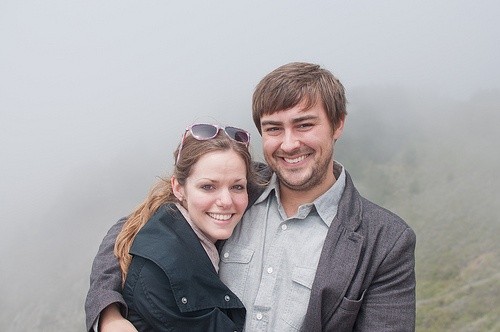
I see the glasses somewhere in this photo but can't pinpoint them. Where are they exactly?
[177,123,250,163]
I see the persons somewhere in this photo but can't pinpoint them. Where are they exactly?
[84,61,416,332]
[113,128,274,332]
